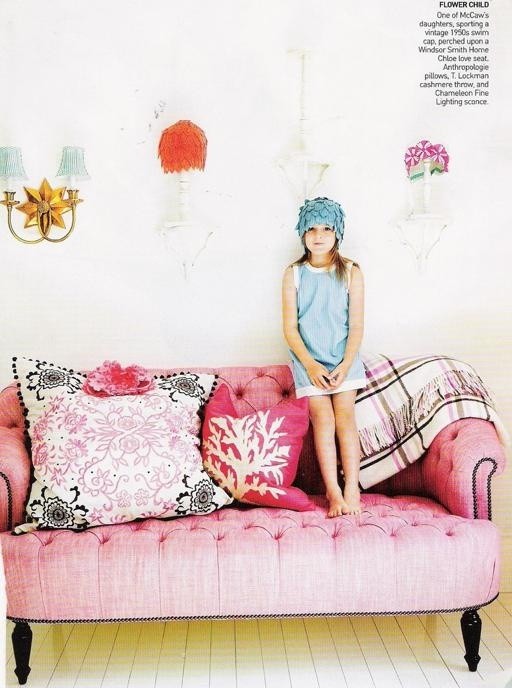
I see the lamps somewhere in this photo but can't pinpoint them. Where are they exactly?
[1,146,92,245]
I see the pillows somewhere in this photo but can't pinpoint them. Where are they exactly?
[15,357,235,534]
[199,384,315,513]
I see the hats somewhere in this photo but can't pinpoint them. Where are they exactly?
[293,196,345,241]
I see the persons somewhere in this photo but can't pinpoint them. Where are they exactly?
[279,196,369,520]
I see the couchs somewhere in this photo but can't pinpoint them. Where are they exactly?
[0,363,512,685]
